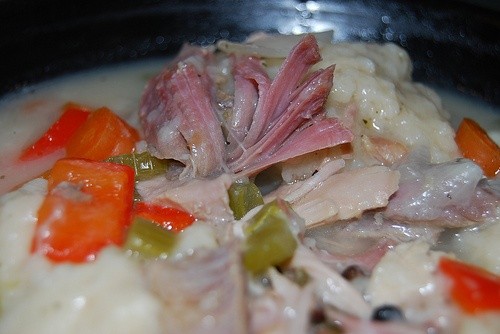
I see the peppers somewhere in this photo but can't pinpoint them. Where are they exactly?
[243,198,294,271]
[43,105,139,181]
[455,117,500,177]
[19,102,93,160]
[134,200,195,234]
[438,256,500,316]
[228,182,263,220]
[30,157,133,264]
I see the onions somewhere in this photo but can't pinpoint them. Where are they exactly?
[0,147,65,194]
